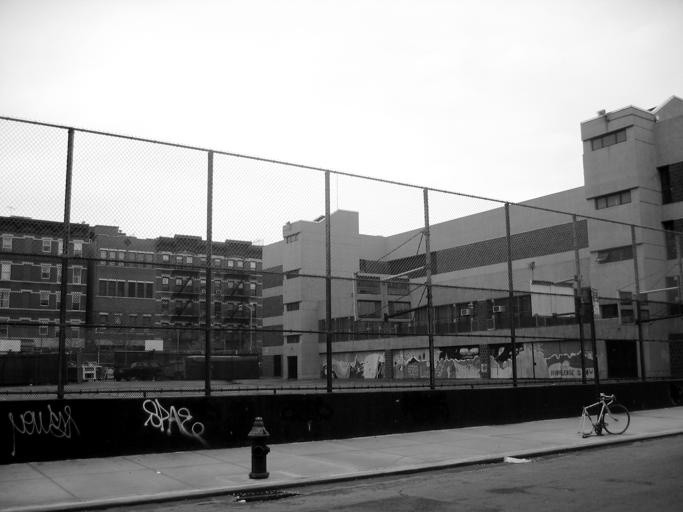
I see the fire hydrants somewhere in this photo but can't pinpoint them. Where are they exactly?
[247,415,273,480]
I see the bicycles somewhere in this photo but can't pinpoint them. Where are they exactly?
[578,391,630,439]
